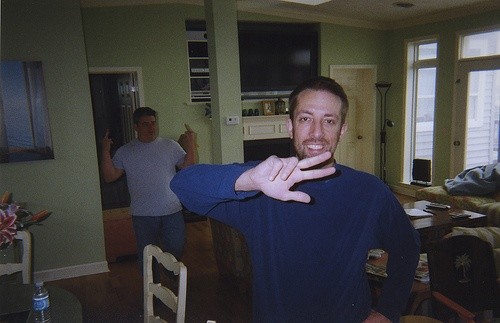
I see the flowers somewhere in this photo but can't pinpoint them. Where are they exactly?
[0,191,50,251]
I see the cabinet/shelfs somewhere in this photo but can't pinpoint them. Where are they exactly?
[187,40,211,103]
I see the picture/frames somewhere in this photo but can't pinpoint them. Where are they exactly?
[261,100,275,115]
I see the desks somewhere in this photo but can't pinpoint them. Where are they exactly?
[0,284,83,323]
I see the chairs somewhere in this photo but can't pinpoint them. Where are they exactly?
[428,235,500,323]
[143,245,188,323]
[0,230,32,285]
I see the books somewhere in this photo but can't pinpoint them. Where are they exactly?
[405,208,432,219]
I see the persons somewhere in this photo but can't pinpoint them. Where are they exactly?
[100,106,199,323]
[168,75,420,323]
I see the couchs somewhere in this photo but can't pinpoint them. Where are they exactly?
[366,186,500,289]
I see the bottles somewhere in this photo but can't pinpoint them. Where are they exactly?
[275,97,286,114]
[32,280,52,323]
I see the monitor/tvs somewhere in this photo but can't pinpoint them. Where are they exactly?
[237,20,318,97]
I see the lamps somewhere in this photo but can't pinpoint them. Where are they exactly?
[375,80,394,184]
[275,97,286,115]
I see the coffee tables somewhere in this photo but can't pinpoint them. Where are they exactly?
[401,200,487,242]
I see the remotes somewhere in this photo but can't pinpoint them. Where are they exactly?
[453,213,471,219]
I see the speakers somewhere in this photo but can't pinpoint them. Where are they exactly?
[412,159,431,182]
